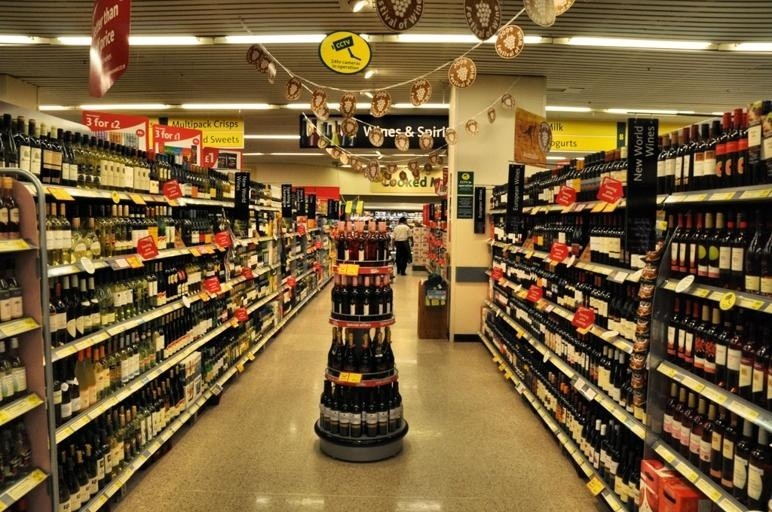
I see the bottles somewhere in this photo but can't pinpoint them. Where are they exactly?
[318,219,403,437]
[663,209,771,296]
[660,99,772,195]
[0,112,318,512]
[483,135,658,511]
[662,289,771,414]
[660,374,771,512]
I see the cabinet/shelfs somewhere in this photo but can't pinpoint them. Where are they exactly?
[643,184,772,512]
[476,193,666,512]
[0,165,59,512]
[17,179,284,512]
[280,228,332,315]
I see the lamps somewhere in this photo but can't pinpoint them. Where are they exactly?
[604,107,696,117]
[719,41,772,56]
[546,104,594,114]
[365,68,377,82]
[364,91,375,99]
[0,33,52,48]
[212,32,382,45]
[352,0,374,14]
[282,101,380,112]
[76,104,174,112]
[563,36,722,52]
[271,153,327,156]
[240,153,265,158]
[382,33,552,47]
[389,102,454,110]
[38,104,77,112]
[180,101,279,110]
[47,34,213,46]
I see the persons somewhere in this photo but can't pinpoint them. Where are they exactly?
[391,217,413,276]
